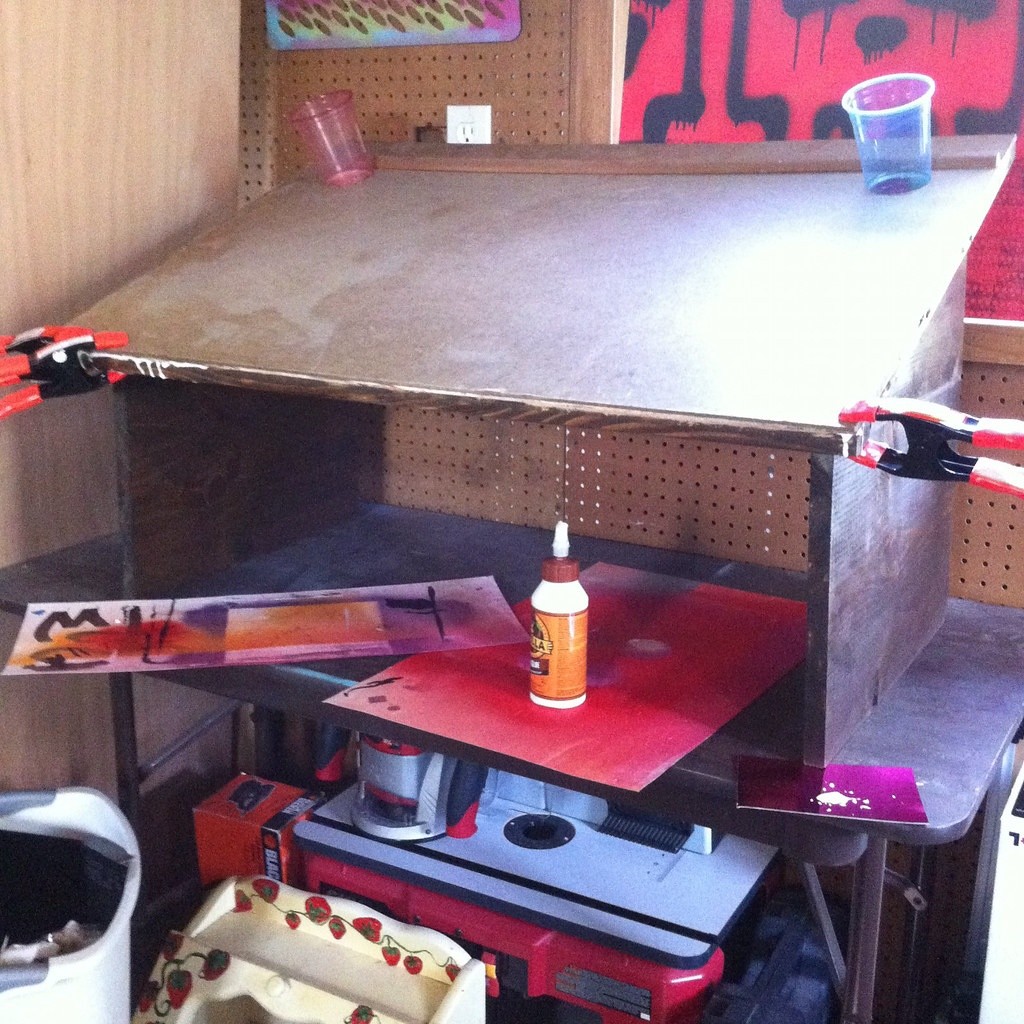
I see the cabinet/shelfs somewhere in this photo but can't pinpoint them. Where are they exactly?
[1,142,1024,1024]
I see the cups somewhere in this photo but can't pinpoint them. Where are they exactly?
[840,73,935,194]
[292,90,375,184]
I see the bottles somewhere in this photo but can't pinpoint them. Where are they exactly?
[528,521,589,709]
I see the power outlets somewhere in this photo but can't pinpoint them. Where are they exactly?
[445,104,492,145]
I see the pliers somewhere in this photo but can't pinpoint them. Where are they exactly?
[0,323,127,414]
[837,397,1024,498]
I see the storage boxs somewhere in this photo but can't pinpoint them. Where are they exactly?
[192,769,847,1024]
